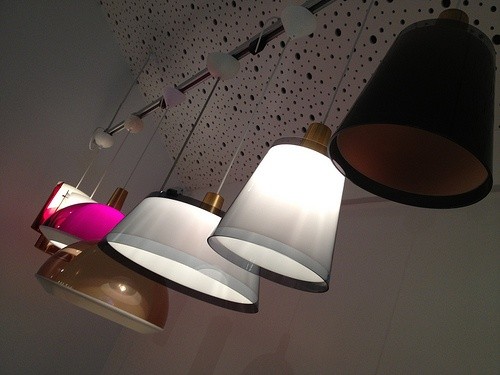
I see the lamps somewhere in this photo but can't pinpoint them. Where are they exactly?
[35,114,143,262]
[208,1,377,293]
[36,52,241,335]
[326,1,496,209]
[31,129,115,234]
[38,84,186,249]
[106,5,319,314]
